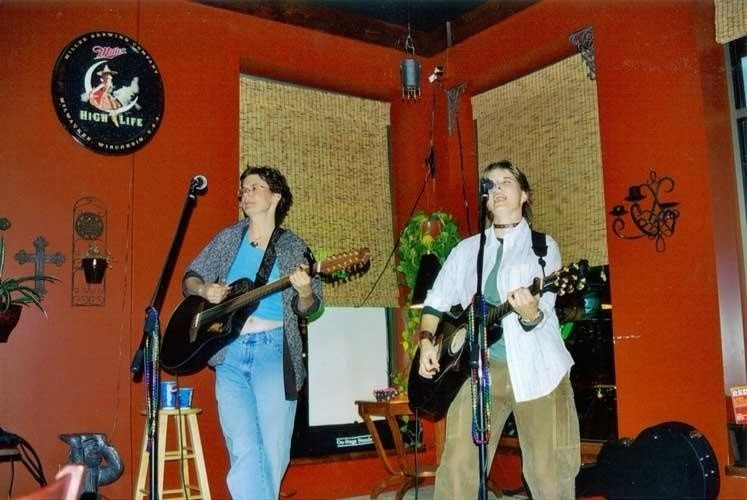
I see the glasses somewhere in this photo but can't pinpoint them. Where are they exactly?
[237,184,271,197]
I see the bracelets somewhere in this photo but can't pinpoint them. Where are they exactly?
[517,308,544,326]
[418,330,434,341]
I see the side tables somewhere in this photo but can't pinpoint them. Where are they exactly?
[354,395,504,500]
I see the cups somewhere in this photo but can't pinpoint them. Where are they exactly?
[159,380,178,410]
[178,388,194,410]
[81,257,108,284]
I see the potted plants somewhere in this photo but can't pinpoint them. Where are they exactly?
[81,241,109,284]
[388,207,472,449]
[0,237,62,342]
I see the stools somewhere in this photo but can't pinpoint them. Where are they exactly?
[133,408,211,500]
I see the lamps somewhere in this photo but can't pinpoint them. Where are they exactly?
[409,65,444,309]
[609,170,681,252]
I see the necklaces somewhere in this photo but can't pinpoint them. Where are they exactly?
[492,222,521,230]
[249,227,272,247]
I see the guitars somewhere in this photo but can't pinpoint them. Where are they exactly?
[159,247,370,377]
[408,258,590,422]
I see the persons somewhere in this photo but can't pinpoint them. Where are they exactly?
[418,159,582,500]
[182,163,325,499]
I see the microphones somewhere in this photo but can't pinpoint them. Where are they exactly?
[482,178,498,193]
[190,175,207,189]
